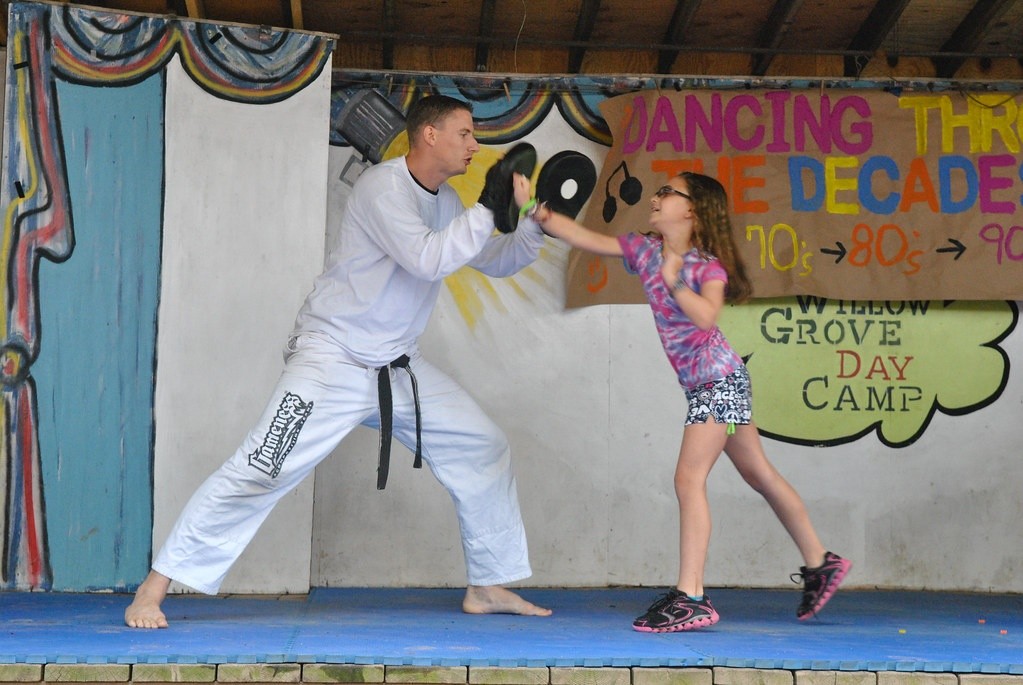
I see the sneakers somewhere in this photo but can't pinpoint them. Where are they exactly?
[789,550,851,619]
[632,587,720,633]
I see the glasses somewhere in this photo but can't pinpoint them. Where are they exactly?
[654,186,692,201]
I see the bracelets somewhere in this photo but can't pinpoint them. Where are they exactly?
[668,278,686,296]
[519,198,552,225]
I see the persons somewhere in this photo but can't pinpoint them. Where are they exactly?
[124,95,553,628]
[512,173,853,633]
